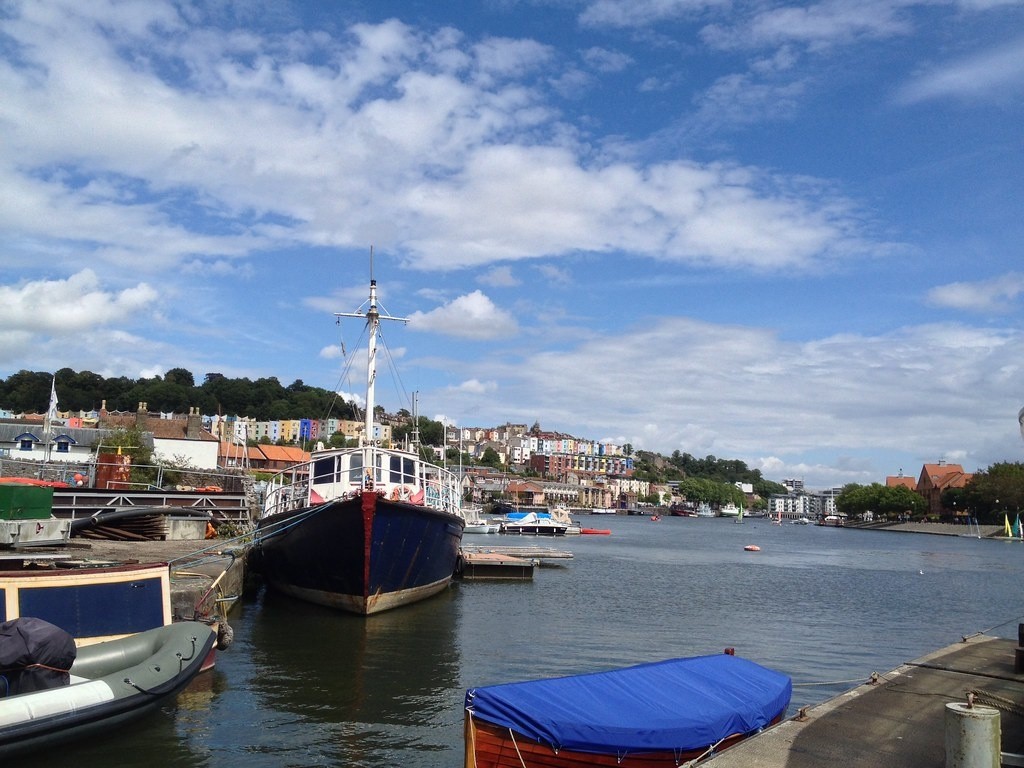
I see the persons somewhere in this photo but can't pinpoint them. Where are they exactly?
[859,515,873,521]
[954,516,976,525]
[921,517,927,523]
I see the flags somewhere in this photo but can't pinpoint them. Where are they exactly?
[47,378,59,424]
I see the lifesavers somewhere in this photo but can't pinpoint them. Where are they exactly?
[206,486,223,492]
[393,485,414,503]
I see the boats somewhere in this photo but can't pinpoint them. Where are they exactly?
[670,499,808,525]
[0,621,219,763]
[240,244,468,617]
[0,561,176,652]
[463,643,793,768]
[463,522,489,534]
[488,523,501,533]
